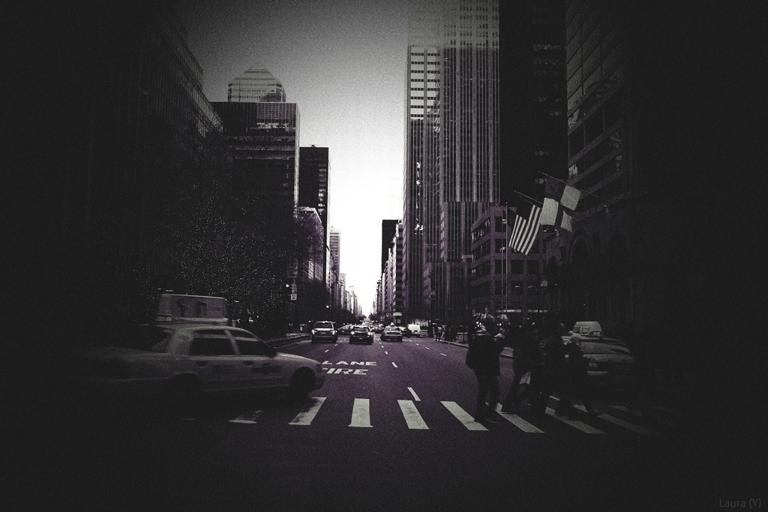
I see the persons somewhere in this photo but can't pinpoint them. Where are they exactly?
[467,314,504,423]
[427,322,458,343]
[500,314,603,417]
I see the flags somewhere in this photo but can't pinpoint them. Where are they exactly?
[509,198,542,254]
[541,174,580,233]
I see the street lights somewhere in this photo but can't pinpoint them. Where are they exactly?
[497,245,504,312]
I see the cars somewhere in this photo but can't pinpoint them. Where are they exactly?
[82,321,331,418]
[537,321,638,397]
[307,317,424,347]
